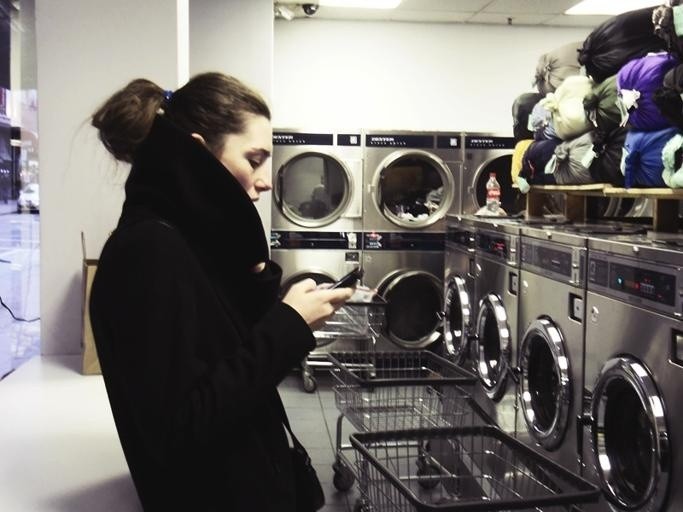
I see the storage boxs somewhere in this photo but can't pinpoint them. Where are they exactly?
[81,230,112,375]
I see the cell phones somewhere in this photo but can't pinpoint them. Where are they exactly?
[328,266,362,290]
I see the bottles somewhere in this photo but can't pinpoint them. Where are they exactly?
[486,173,501,216]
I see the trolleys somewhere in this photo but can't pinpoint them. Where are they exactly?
[302,286,390,393]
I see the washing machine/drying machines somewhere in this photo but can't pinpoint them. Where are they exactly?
[274,131,529,370]
[444,214,681,510]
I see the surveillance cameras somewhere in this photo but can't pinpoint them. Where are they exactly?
[303,4,319,15]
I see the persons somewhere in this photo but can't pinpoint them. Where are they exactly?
[91,72,360,512]
